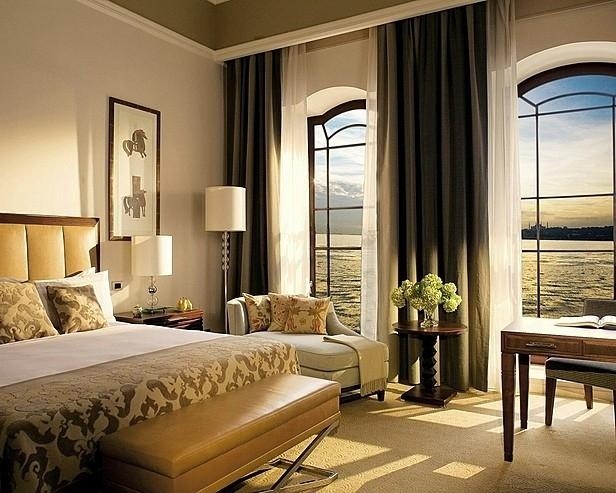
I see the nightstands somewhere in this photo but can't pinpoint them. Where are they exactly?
[115,307,204,331]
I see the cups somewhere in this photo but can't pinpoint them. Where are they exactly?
[132,306,144,317]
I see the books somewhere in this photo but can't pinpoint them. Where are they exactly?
[554,315,616,329]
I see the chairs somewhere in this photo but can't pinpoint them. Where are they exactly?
[545,298,616,427]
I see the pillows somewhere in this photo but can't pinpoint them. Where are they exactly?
[1,267,117,344]
[243,292,332,334]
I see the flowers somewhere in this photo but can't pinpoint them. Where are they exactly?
[391,273,462,319]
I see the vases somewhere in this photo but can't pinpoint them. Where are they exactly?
[420,310,440,329]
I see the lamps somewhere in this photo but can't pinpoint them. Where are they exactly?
[131,235,173,313]
[205,186,247,334]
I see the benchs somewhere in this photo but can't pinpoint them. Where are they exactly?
[227,295,389,405]
[100,372,341,493]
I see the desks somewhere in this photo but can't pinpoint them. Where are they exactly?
[501,317,616,462]
[392,320,467,408]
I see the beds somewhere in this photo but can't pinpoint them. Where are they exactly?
[0,213,302,493]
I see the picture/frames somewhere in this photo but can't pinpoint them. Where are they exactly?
[108,96,160,242]
